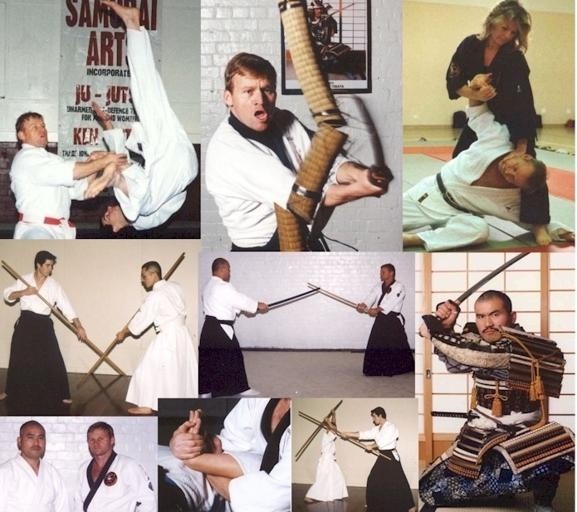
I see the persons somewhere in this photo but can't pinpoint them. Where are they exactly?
[402,71,575,253]
[0,250,87,405]
[114,261,198,415]
[205,52,385,252]
[84,1,198,239]
[446,0,553,250]
[357,263,416,378]
[420,290,575,512]
[158,407,265,511]
[303,408,349,503]
[8,111,128,239]
[0,420,64,512]
[64,421,157,512]
[340,406,416,512]
[198,257,268,398]
[168,397,292,511]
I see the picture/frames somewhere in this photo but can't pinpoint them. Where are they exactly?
[280,0,371,95]
